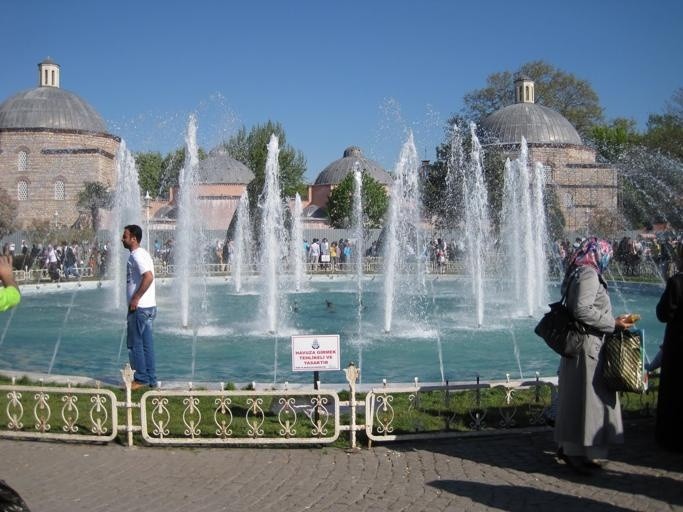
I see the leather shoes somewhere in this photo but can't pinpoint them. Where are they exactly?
[120,380,144,390]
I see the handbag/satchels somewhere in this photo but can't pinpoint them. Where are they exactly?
[599,327,651,392]
[537,302,586,357]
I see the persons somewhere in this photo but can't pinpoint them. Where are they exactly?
[553,237,637,470]
[121,225,158,391]
[0,254,22,314]
[645,342,664,371]
[0,222,683,285]
[653,240,682,453]
[0,481,29,510]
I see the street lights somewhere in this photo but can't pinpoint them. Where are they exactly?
[141,190,152,252]
[53,210,59,227]
